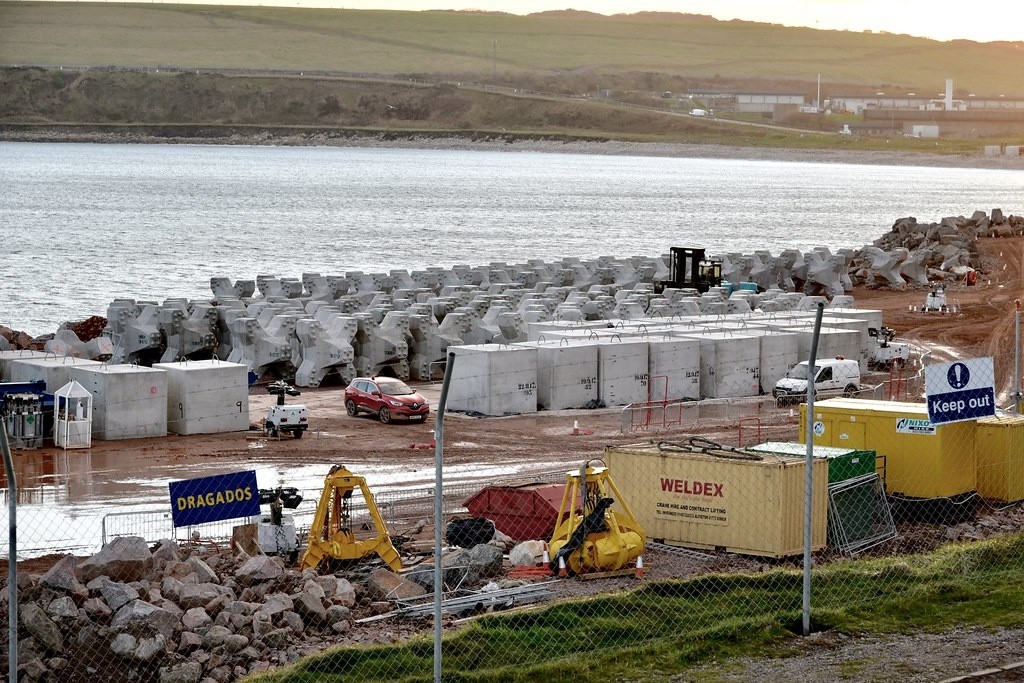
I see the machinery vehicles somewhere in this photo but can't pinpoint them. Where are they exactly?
[924,281,950,315]
[264,381,310,438]
[868,326,911,372]
[651,245,724,293]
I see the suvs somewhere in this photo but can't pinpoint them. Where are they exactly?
[344,375,429,425]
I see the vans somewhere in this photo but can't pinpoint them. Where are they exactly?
[770,354,863,408]
